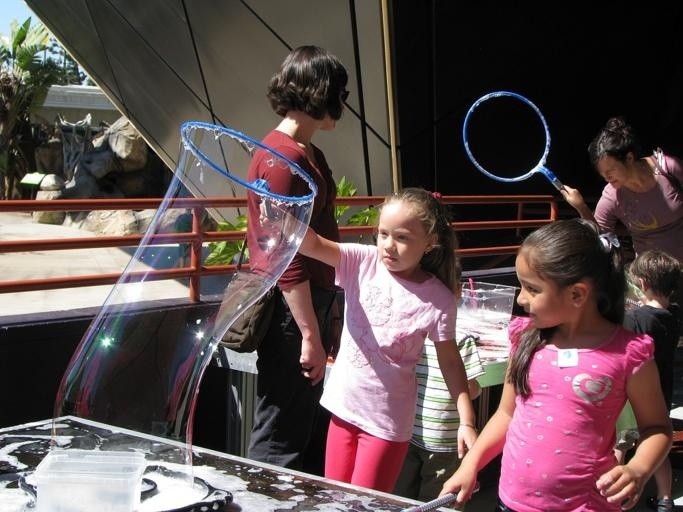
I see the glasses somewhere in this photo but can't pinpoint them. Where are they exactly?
[341,88,351,102]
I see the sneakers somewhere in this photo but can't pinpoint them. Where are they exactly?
[648,496,674,511]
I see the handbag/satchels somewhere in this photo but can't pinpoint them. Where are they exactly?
[211,271,276,353]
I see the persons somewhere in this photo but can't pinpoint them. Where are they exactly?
[245,44,345,475]
[559,184,600,231]
[623,247,683,512]
[587,116,682,266]
[393,257,485,508]
[257,186,478,494]
[437,216,674,512]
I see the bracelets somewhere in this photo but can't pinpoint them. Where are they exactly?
[333,316,342,321]
[458,423,476,430]
